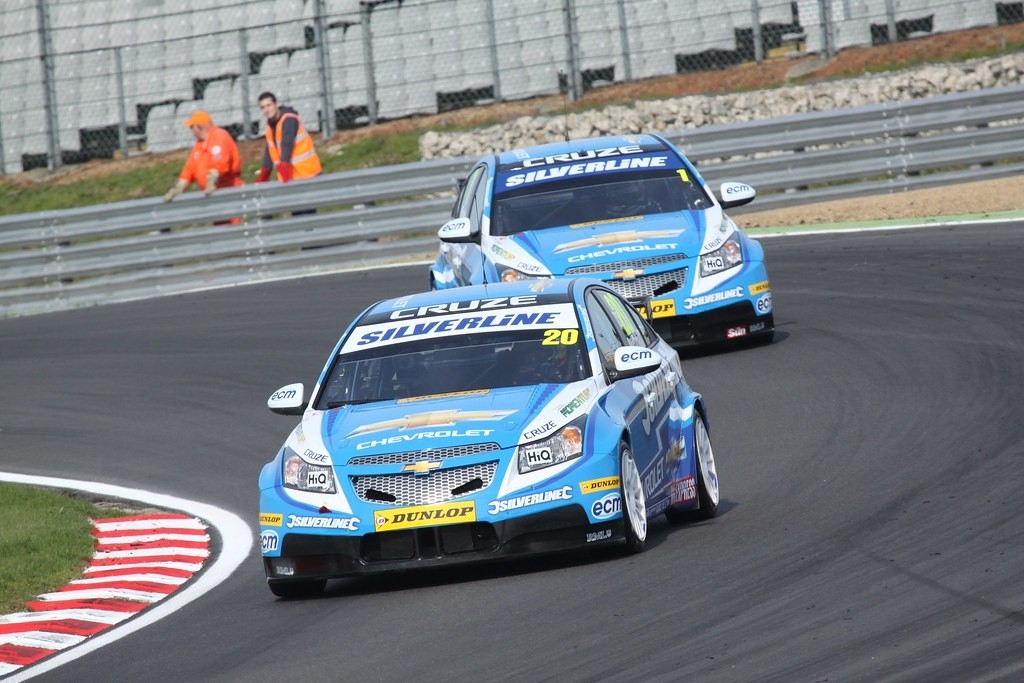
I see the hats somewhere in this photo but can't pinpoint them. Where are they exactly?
[183,112,211,126]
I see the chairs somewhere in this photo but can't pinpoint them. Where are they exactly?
[0,0,1023,173]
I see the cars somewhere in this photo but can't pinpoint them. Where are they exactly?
[427,132,777,356]
[257,275,720,601]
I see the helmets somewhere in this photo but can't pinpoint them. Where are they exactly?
[605,175,650,218]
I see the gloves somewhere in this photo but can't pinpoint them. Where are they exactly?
[278,161,294,181]
[254,167,271,184]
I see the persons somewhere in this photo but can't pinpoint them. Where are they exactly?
[605,180,664,219]
[163,110,247,227]
[518,345,581,385]
[253,92,325,250]
